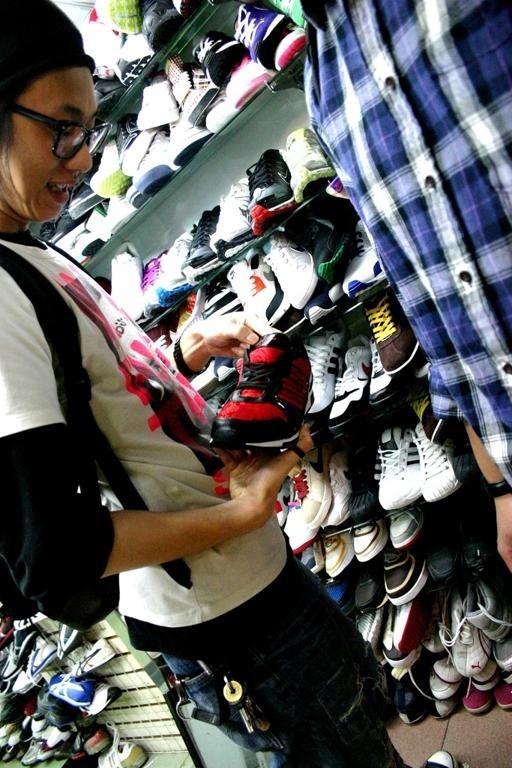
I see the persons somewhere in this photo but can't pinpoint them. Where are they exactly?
[300,0,512,574]
[0,0,458,768]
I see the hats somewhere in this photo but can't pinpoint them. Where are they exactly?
[0,2,85,85]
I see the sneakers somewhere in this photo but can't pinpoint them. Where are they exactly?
[154,233,194,309]
[144,257,163,321]
[422,750,459,768]
[354,584,512,724]
[306,295,434,437]
[179,291,195,330]
[225,260,289,332]
[295,506,489,614]
[345,219,387,297]
[247,149,297,238]
[232,0,307,72]
[277,128,336,202]
[405,380,460,443]
[192,29,277,110]
[275,442,377,552]
[264,233,317,311]
[181,205,221,285]
[118,54,240,208]
[410,418,461,504]
[68,154,108,219]
[211,175,257,264]
[374,424,422,511]
[109,241,143,326]
[448,416,485,489]
[306,187,359,285]
[91,116,138,199]
[211,330,316,458]
[0,611,151,768]
[202,289,251,379]
[44,208,74,244]
[67,0,181,81]
[307,287,342,324]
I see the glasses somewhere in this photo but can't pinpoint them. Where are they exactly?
[5,101,114,161]
